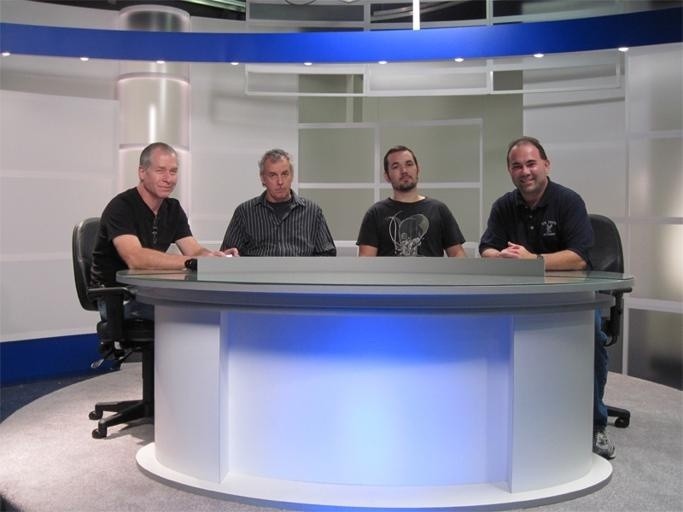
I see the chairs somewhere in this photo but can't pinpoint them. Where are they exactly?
[73,218,154,438]
[589,215,629,428]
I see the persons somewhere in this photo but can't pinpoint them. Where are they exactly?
[91,142,240,320]
[219,149,336,256]
[355,146,467,257]
[478,137,617,459]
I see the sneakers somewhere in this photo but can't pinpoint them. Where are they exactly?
[593,432,615,459]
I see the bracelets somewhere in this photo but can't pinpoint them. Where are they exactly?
[537,254,544,260]
